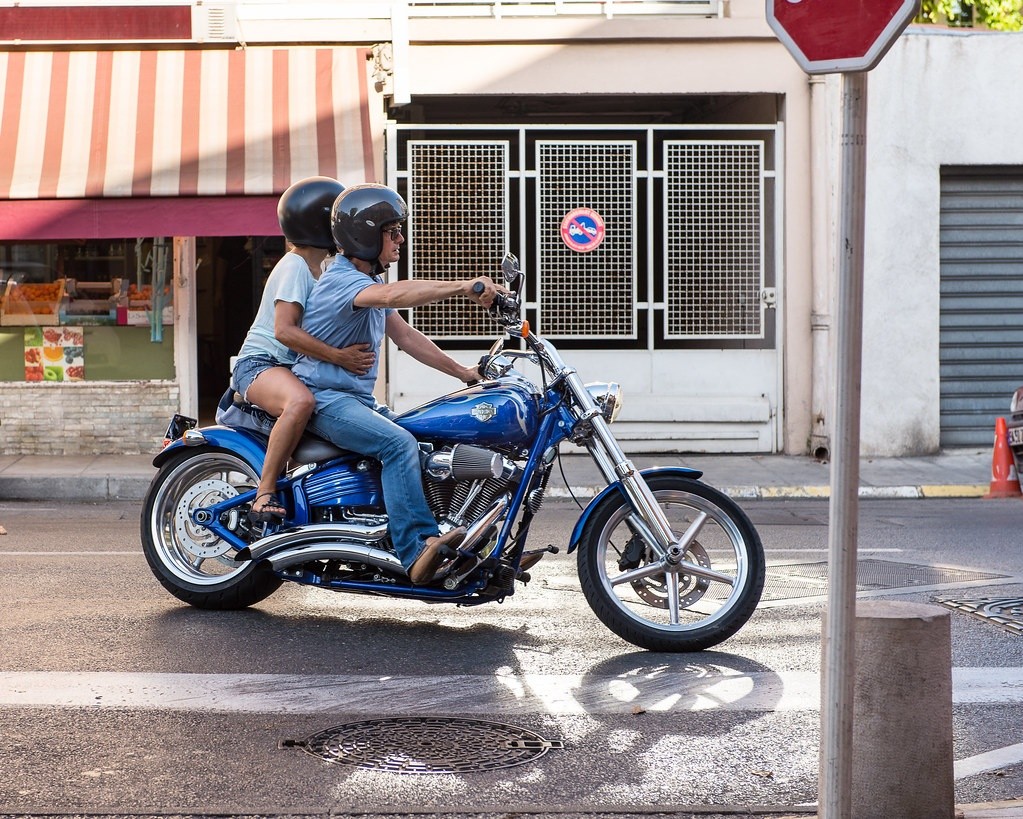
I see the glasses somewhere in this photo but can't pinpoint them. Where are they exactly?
[383,225,402,241]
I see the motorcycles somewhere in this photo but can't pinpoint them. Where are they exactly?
[137,252,767,653]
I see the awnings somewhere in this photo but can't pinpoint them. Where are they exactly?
[0,47,380,241]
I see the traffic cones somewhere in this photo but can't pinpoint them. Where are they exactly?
[980,418,1022,500]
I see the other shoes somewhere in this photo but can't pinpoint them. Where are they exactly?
[517,551,545,571]
[410,526,467,586]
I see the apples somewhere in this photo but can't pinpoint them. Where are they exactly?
[7,283,61,314]
[44,366,63,381]
[128,283,175,310]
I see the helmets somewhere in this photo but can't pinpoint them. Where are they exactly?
[276,176,348,257]
[331,183,409,275]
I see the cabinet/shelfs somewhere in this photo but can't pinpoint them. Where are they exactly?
[0,241,130,300]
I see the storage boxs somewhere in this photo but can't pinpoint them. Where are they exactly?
[0,276,173,325]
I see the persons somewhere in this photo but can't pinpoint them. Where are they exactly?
[214,237,254,359]
[290,184,509,587]
[232,177,377,523]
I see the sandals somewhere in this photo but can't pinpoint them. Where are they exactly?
[249,492,287,518]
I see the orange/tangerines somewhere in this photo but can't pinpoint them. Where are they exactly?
[44,347,63,361]
